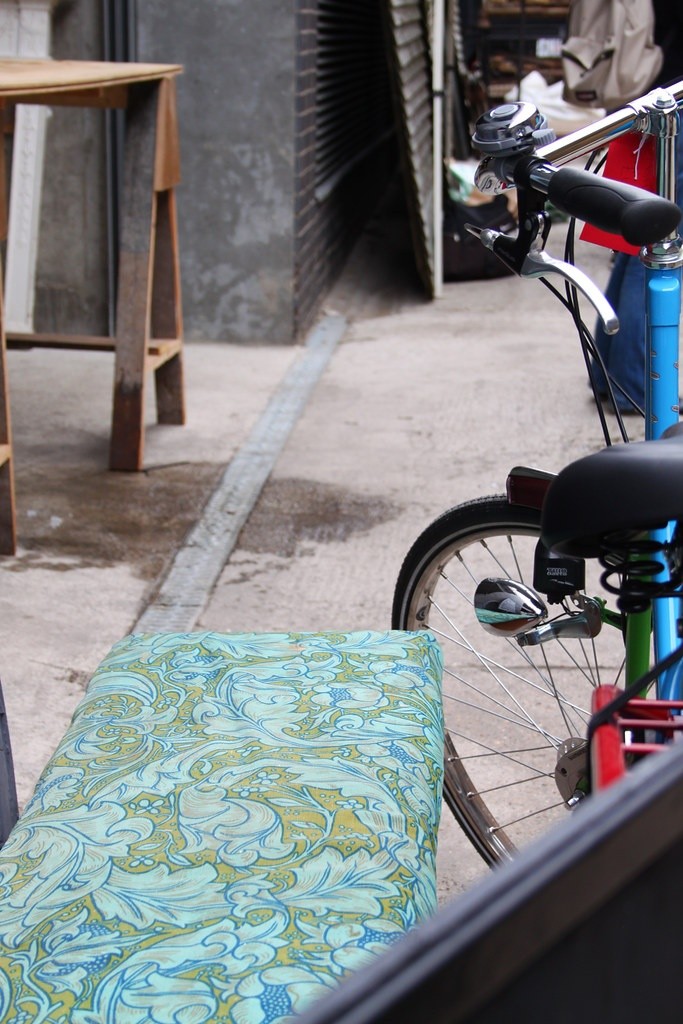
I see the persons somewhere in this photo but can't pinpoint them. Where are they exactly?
[579,0,682,417]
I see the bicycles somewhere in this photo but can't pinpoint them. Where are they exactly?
[388,75,683,876]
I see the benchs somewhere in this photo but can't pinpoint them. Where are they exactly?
[0,629,444,1023]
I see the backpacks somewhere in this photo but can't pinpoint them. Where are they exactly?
[560,0,663,110]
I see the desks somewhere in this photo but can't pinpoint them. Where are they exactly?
[0,57,189,559]
